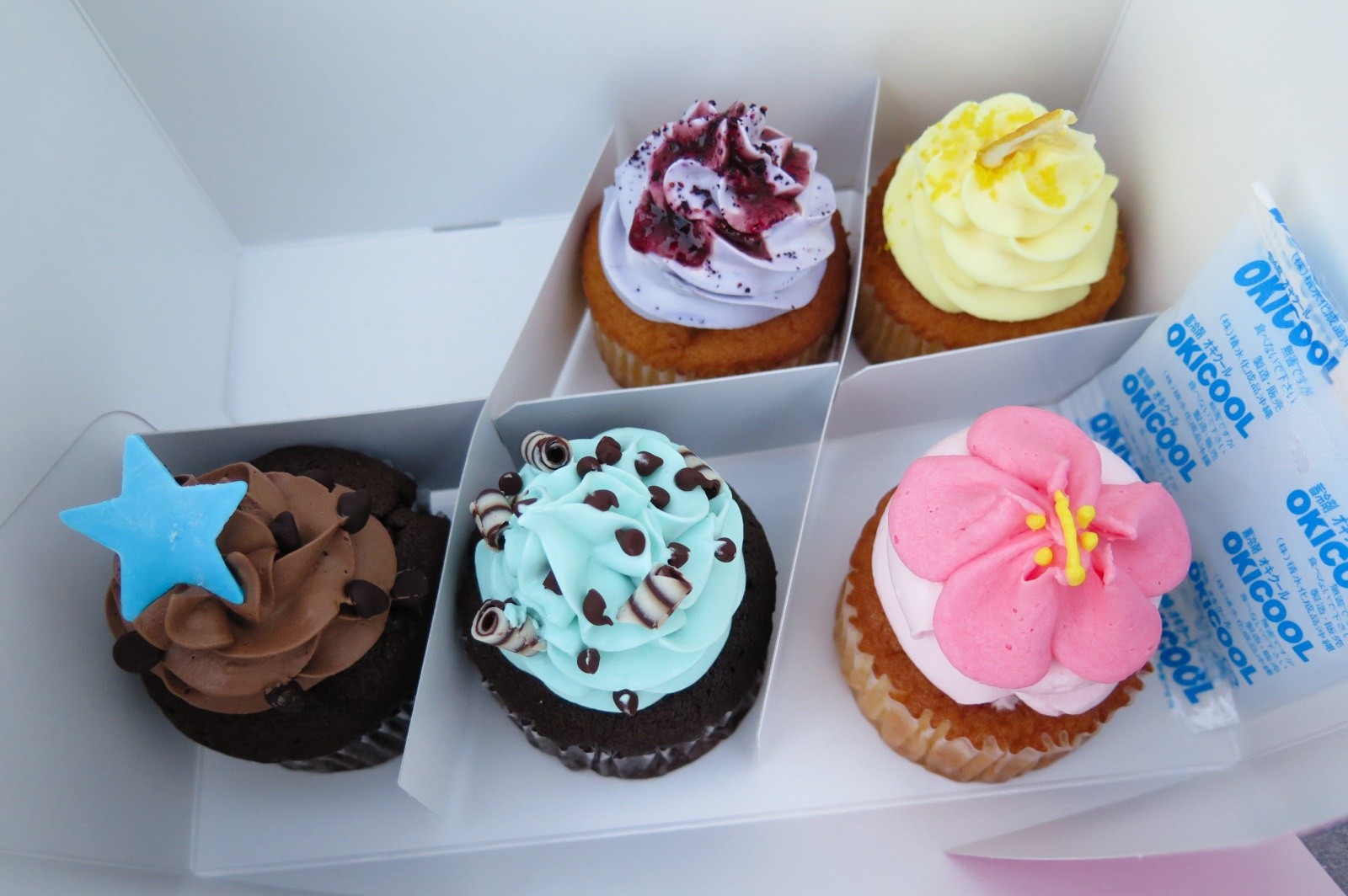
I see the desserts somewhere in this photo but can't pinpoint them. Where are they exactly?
[833,404,1194,783]
[461,423,778,779]
[853,89,1132,367]
[57,433,450,774]
[580,95,852,391]
